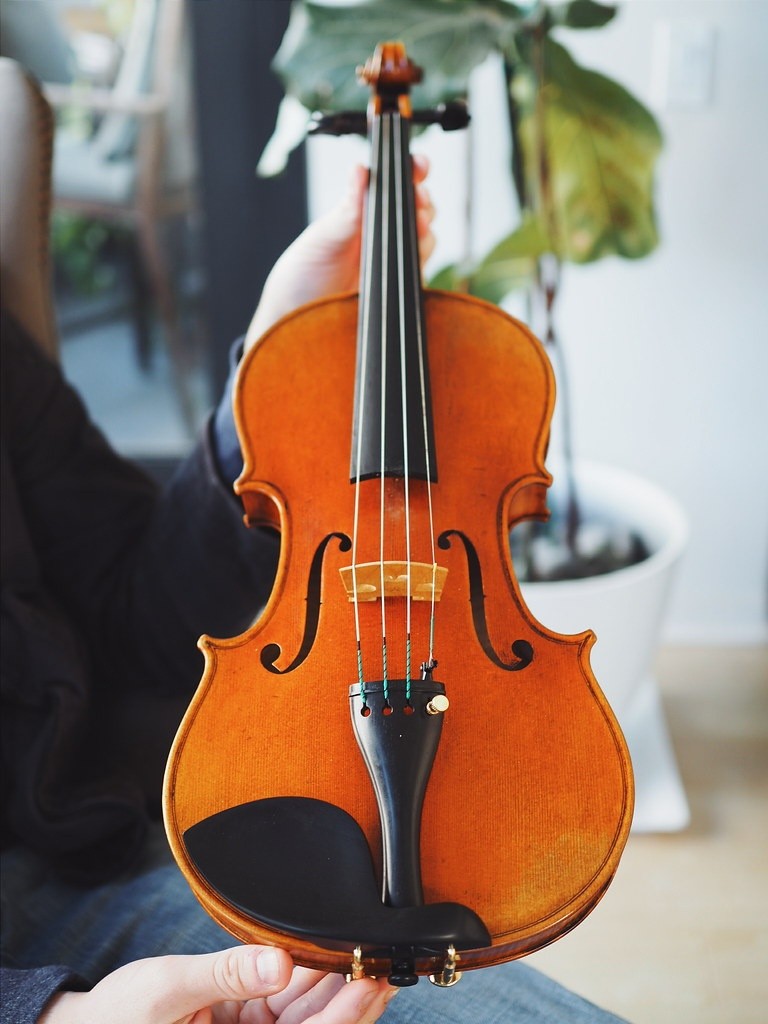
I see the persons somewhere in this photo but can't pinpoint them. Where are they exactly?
[0,151,624,1024]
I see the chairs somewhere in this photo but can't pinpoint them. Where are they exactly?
[43,2,197,385]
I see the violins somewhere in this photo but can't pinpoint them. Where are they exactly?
[157,38,639,990]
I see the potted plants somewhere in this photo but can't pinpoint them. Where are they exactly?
[258,0,691,836]
